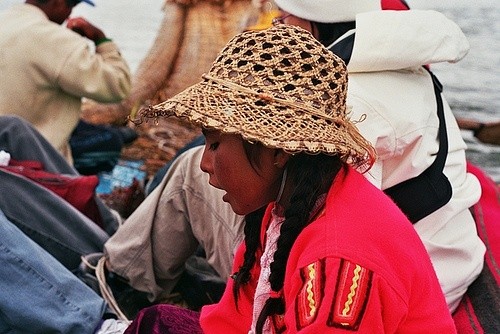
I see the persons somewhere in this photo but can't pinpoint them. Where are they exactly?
[1,0,488,334]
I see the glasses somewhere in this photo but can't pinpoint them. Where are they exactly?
[272,14,292,27]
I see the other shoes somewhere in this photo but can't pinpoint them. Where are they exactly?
[96,318,132,334]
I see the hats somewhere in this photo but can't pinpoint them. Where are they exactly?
[136,21,377,173]
